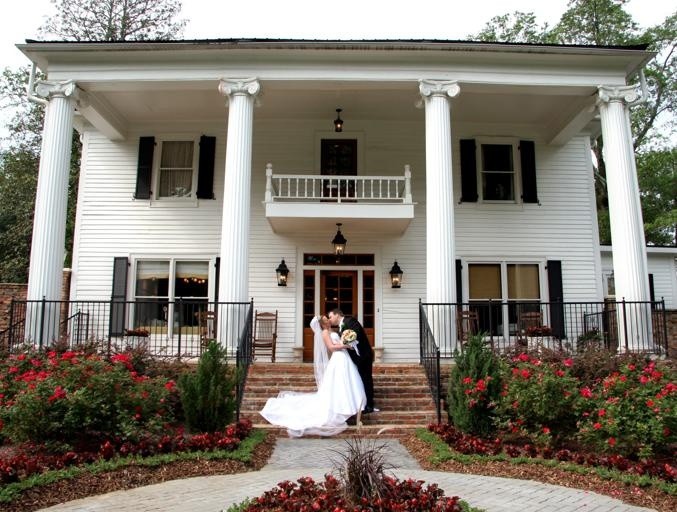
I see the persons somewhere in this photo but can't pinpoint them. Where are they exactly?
[328,308,376,412]
[309,312,366,421]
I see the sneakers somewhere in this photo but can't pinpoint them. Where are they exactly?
[362,407,374,414]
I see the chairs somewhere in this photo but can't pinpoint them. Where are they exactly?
[251,309,277,362]
[455,308,495,354]
[194,311,217,360]
[515,310,543,346]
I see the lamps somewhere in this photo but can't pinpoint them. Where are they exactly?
[333,109,343,132]
[330,223,347,260]
[274,257,289,286]
[389,258,403,288]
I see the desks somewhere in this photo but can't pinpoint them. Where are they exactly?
[526,333,555,353]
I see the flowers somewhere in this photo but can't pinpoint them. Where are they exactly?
[341,321,347,329]
[526,325,552,333]
[339,330,360,357]
